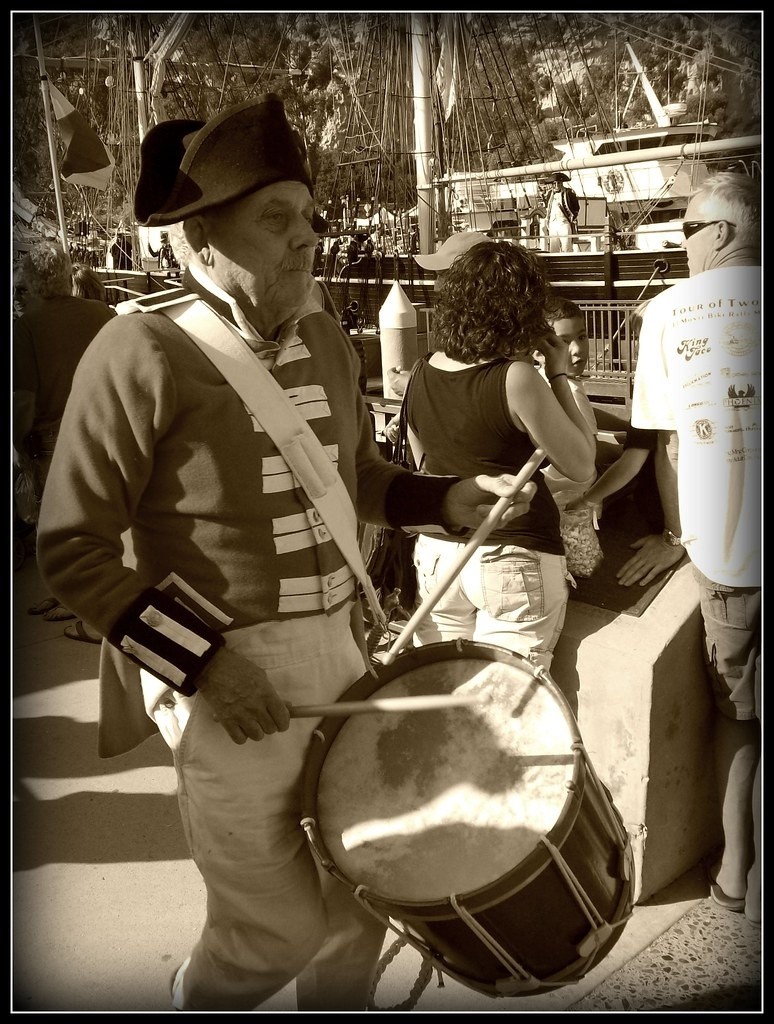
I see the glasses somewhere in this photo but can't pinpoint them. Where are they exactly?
[552,182,561,185]
[683,219,737,240]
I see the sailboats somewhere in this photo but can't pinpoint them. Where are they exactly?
[31,10,761,343]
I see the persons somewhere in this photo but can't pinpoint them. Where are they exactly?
[39,88,539,1010]
[148,231,182,284]
[11,234,131,645]
[384,172,761,921]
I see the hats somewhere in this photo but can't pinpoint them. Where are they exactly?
[412,231,494,271]
[133,92,330,234]
[545,173,571,183]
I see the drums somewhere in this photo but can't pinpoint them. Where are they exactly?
[293,636,637,996]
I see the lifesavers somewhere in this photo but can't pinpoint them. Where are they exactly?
[604,169,627,195]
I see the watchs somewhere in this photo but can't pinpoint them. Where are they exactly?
[661,528,682,546]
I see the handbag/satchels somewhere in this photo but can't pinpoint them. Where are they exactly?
[97,637,160,760]
[14,462,39,525]
[356,354,424,624]
[552,489,604,578]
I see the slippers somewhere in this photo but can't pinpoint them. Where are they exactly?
[43,603,77,621]
[63,620,103,644]
[745,904,761,928]
[28,597,61,616]
[702,849,745,911]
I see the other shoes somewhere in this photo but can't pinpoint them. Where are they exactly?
[169,956,192,1011]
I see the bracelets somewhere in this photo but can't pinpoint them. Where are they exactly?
[580,491,597,507]
[548,372,566,382]
